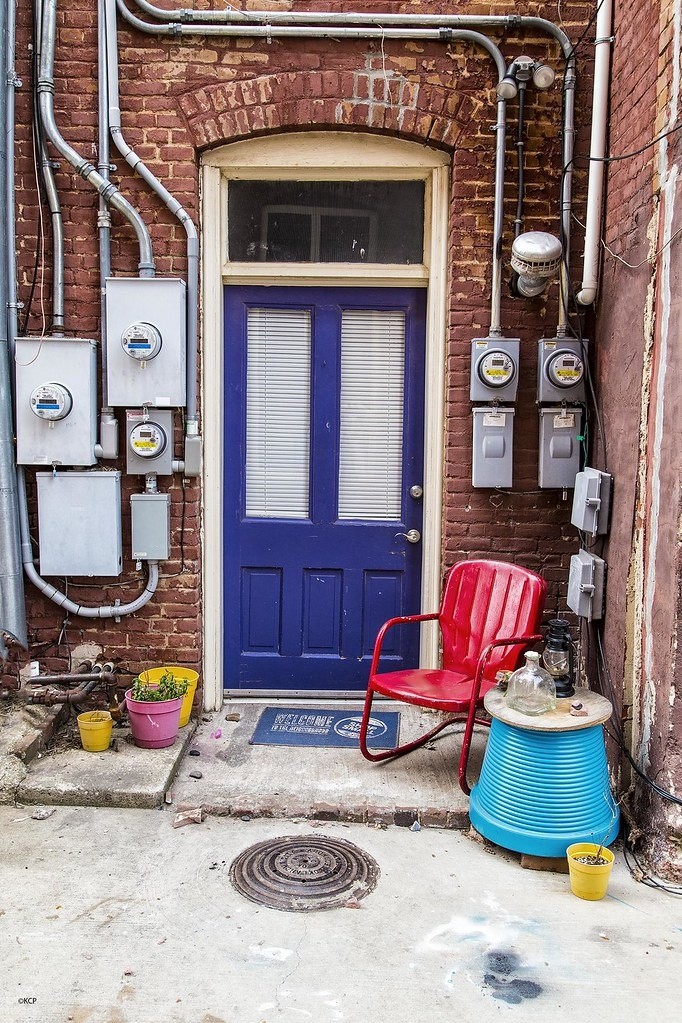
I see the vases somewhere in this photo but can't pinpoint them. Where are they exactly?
[567,842,615,901]
[139,666,199,727]
[77,711,112,752]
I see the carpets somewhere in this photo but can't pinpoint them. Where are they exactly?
[250,707,401,749]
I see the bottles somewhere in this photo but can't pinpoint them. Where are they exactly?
[542,619,575,698]
[507,650,556,717]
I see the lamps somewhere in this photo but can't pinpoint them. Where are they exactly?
[542,619,576,698]
[496,62,519,99]
[532,62,555,88]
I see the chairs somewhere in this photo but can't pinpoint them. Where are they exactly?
[359,559,546,796]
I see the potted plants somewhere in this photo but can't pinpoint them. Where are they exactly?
[125,669,191,748]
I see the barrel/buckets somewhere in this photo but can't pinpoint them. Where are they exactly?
[138,667,199,729]
